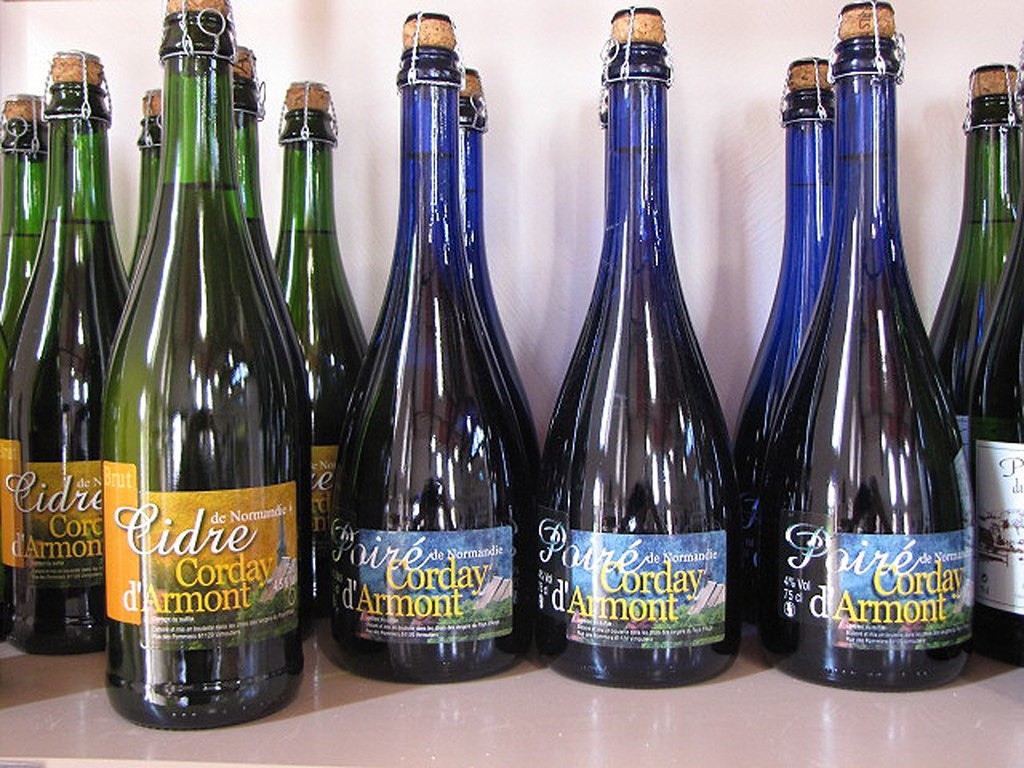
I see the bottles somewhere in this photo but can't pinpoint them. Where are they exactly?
[0,3,1024,730]
